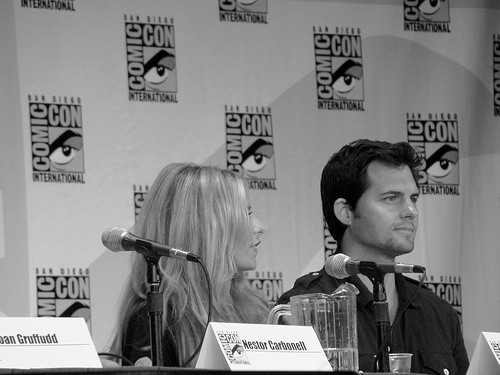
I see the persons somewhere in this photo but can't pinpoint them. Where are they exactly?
[106,163,271,367]
[273,140,470,375]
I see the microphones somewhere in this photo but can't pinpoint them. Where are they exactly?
[325,254,426,279]
[101,225,201,264]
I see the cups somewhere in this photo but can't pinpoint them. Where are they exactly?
[388,352,413,373]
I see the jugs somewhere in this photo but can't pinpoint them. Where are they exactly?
[267,281,359,372]
